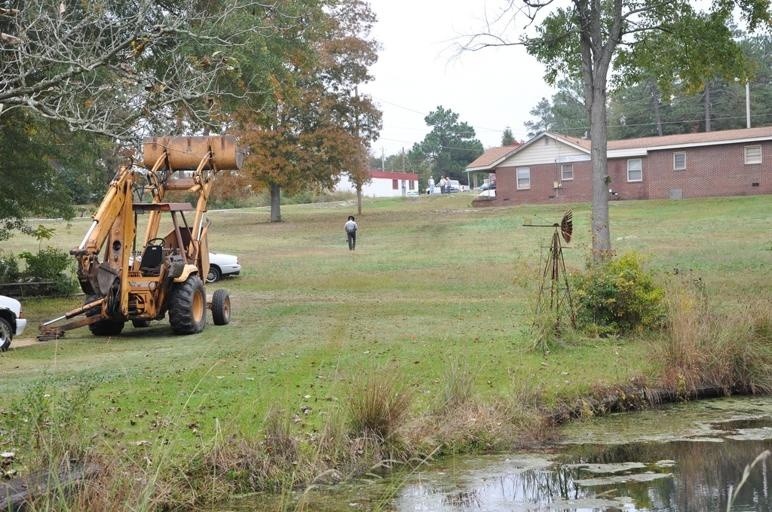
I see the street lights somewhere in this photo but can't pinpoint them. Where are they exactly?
[734,76,752,129]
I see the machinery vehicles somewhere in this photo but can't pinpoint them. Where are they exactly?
[37,133,253,343]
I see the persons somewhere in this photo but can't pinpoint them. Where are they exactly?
[345,216,358,251]
[439,176,446,193]
[428,176,435,194]
[445,177,451,194]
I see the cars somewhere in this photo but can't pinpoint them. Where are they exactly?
[426,179,461,193]
[1,294,28,353]
[206,252,240,283]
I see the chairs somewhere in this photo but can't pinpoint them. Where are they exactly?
[140,246,163,275]
